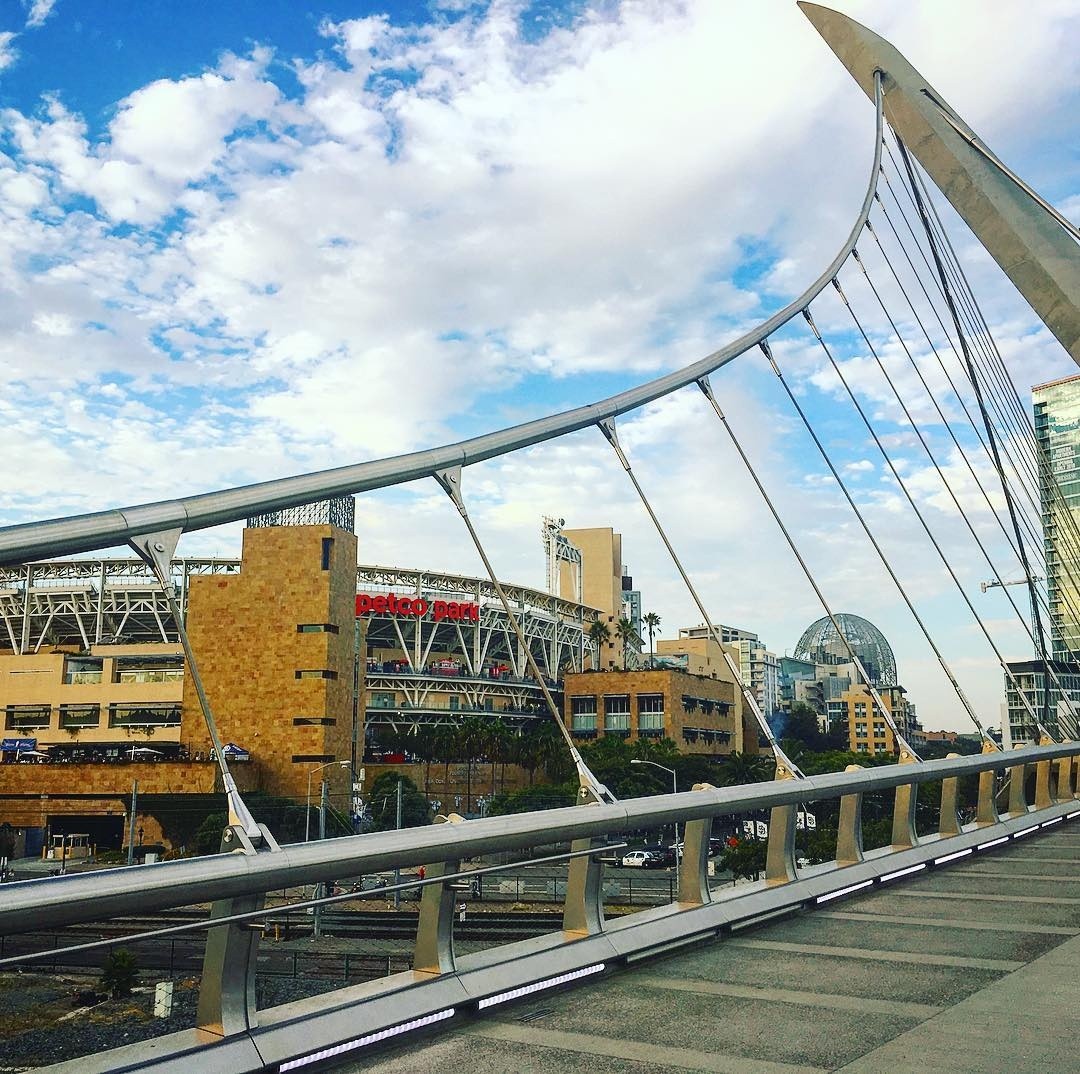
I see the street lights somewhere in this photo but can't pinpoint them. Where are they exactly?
[302,761,351,900]
[630,760,680,893]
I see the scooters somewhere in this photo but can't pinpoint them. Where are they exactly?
[349,875,389,901]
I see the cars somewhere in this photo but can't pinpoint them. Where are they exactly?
[634,844,674,864]
[669,837,725,863]
[613,851,659,869]
